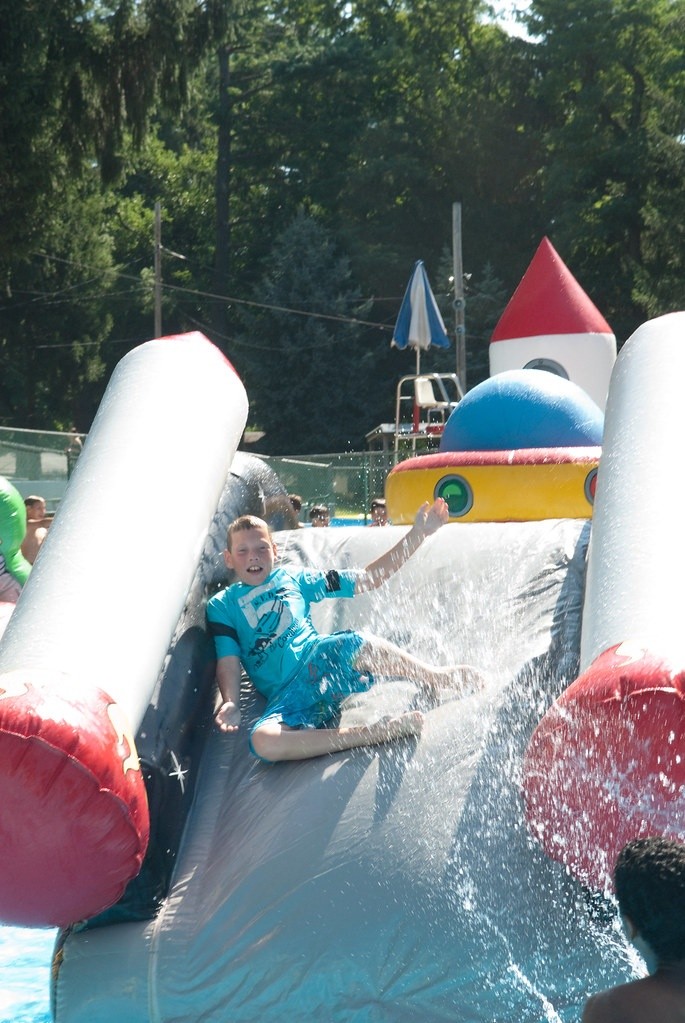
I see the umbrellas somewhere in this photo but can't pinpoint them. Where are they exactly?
[390,260,450,375]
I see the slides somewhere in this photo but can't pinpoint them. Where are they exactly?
[0,307,681,1023]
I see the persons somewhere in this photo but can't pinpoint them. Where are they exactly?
[64,428,82,466]
[289,495,301,515]
[370,498,388,527]
[206,497,486,765]
[21,495,54,565]
[578,836,685,1023]
[311,506,328,527]
